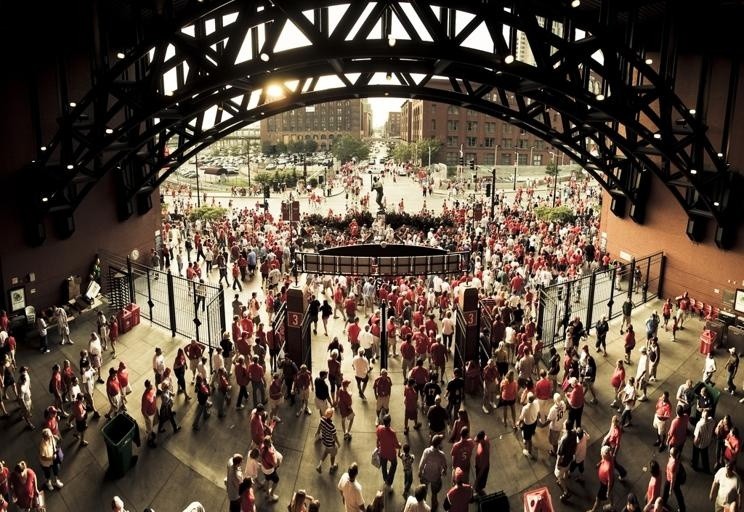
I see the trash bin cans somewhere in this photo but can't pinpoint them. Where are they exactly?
[102,412,140,473]
[700,329,717,354]
[477,490,510,512]
[126,303,141,325]
[523,487,555,512]
[117,308,131,331]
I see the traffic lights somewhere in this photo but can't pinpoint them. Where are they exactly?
[485,184,491,197]
[264,185,269,198]
[470,160,474,170]
[460,156,463,166]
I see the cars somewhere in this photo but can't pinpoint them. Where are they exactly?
[357,138,409,176]
[174,147,336,180]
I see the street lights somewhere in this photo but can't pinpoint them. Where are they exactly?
[240,136,250,188]
[515,152,519,174]
[530,147,536,165]
[549,151,555,163]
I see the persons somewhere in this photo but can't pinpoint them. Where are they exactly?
[1,155,743,511]
